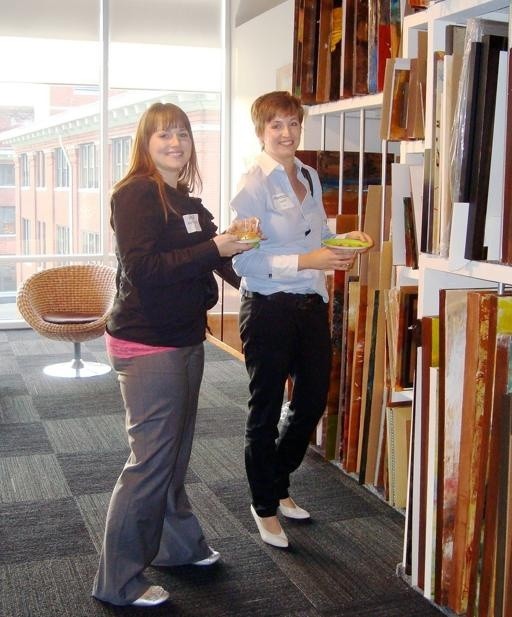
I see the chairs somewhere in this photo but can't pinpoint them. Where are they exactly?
[17,264,117,381]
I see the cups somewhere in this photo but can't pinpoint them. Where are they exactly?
[239,217,259,234]
[335,248,358,271]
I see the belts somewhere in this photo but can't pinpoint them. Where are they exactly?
[244,291,317,310]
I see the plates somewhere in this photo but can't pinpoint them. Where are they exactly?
[232,237,262,245]
[321,238,369,254]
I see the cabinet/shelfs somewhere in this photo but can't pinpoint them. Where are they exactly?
[291,1,510,616]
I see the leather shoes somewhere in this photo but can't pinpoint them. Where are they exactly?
[249,505,288,547]
[279,497,310,519]
[193,551,220,565]
[133,584,169,607]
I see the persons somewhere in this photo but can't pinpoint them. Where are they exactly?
[229,90,375,549]
[90,100,269,607]
